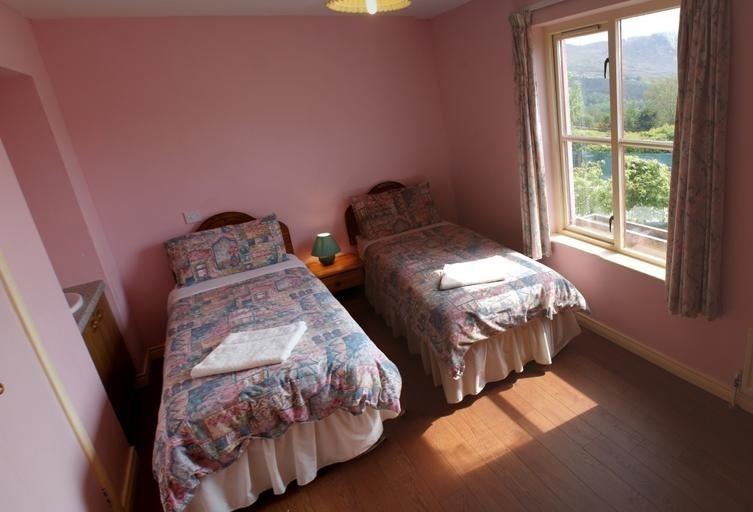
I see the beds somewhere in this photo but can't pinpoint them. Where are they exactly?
[149,211,405,512]
[345,181,591,404]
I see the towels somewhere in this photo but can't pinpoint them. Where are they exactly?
[189,320,309,380]
[436,258,505,289]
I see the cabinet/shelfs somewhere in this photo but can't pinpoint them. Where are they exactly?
[83,292,141,445]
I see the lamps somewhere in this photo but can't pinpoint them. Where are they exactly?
[325,0,413,16]
[310,232,340,266]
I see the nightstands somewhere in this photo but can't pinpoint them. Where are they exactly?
[308,253,366,293]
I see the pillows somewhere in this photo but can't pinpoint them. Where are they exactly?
[162,211,291,290]
[350,182,442,241]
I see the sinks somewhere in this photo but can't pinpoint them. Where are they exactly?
[64,291,85,312]
